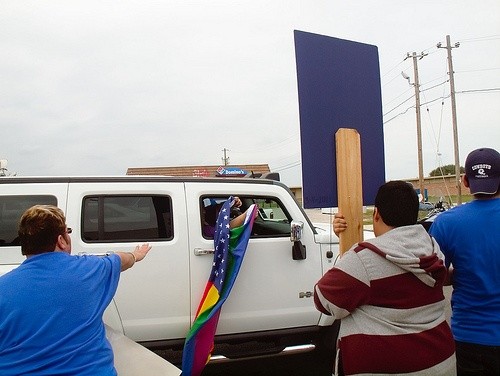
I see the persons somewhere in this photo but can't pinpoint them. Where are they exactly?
[200,196,259,241]
[314,180,458,376]
[0,204,153,376]
[427,148,499,376]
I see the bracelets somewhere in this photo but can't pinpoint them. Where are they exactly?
[128,251,136,267]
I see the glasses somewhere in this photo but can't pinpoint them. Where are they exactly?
[62,228,73,235]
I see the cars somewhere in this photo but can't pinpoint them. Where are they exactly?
[0,174,376,352]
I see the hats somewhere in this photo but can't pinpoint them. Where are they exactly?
[465,148,500,195]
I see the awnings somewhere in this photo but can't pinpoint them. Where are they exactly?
[215,167,248,178]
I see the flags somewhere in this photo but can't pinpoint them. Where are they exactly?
[179,196,260,376]
